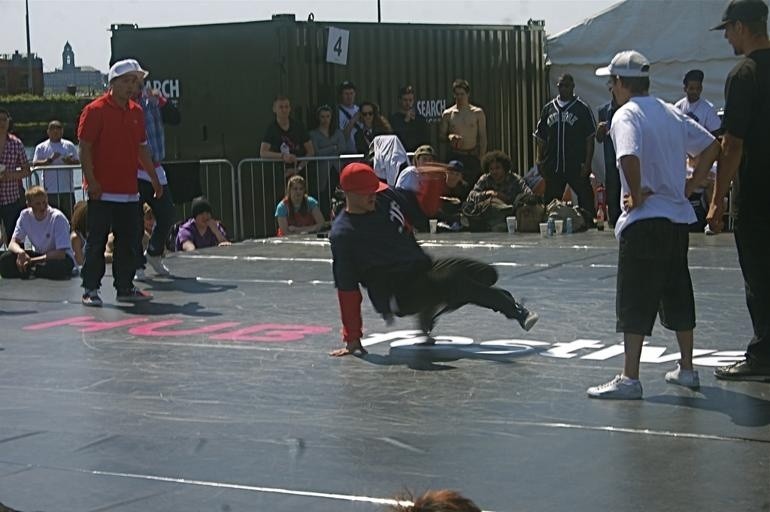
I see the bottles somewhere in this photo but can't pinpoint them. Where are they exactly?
[595,205,606,233]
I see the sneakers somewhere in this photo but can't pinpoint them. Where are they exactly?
[514,300,540,332]
[143,248,171,277]
[712,360,769,382]
[132,268,154,282]
[81,292,103,307]
[586,375,644,400]
[665,359,700,390]
[116,286,154,302]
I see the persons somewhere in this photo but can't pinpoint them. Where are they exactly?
[329,162,540,359]
[707,0,770,385]
[260,65,622,237]
[673,70,722,231]
[77,60,155,307]
[584,49,721,401]
[131,59,177,282]
[1,110,156,280]
[171,196,227,252]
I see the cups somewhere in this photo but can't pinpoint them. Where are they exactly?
[429,218,438,234]
[538,219,574,239]
[506,216,517,234]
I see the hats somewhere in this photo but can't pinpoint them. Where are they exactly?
[190,193,214,219]
[595,51,651,78]
[339,162,390,195]
[412,145,437,165]
[708,1,769,32]
[682,69,705,84]
[108,59,149,86]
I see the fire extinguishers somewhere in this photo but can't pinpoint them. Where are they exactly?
[594,183,606,221]
